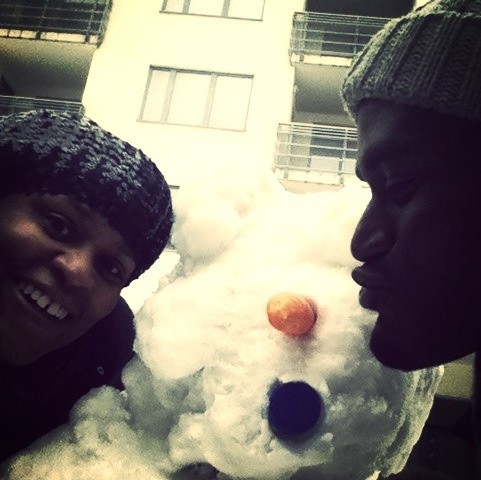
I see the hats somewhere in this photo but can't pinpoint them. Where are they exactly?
[1,106,175,284]
[340,0,480,130]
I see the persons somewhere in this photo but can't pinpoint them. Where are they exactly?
[341,0,481,372]
[0,109,173,461]
